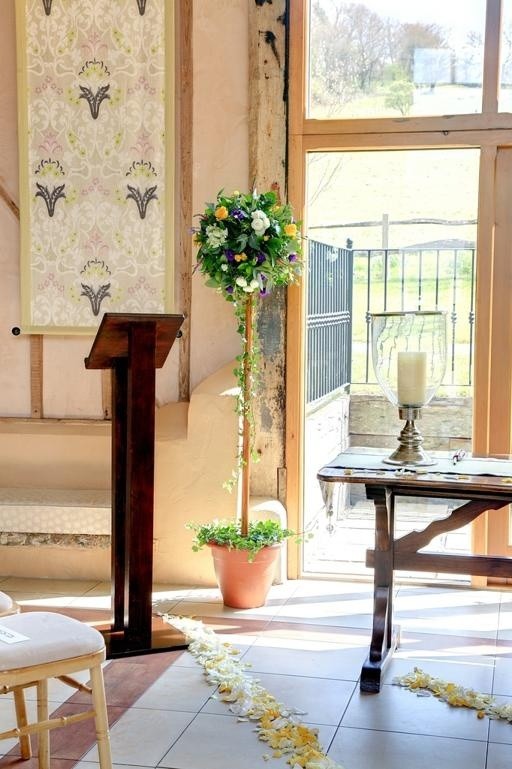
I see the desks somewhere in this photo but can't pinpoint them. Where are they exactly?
[317,445,512,694]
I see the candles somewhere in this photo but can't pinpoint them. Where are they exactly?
[397,349,428,408]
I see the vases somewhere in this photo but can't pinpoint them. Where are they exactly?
[206,539,283,611]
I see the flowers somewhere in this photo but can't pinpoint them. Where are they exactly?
[392,665,512,725]
[188,185,312,565]
[160,611,341,768]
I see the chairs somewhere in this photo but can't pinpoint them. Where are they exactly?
[0,590,113,769]
